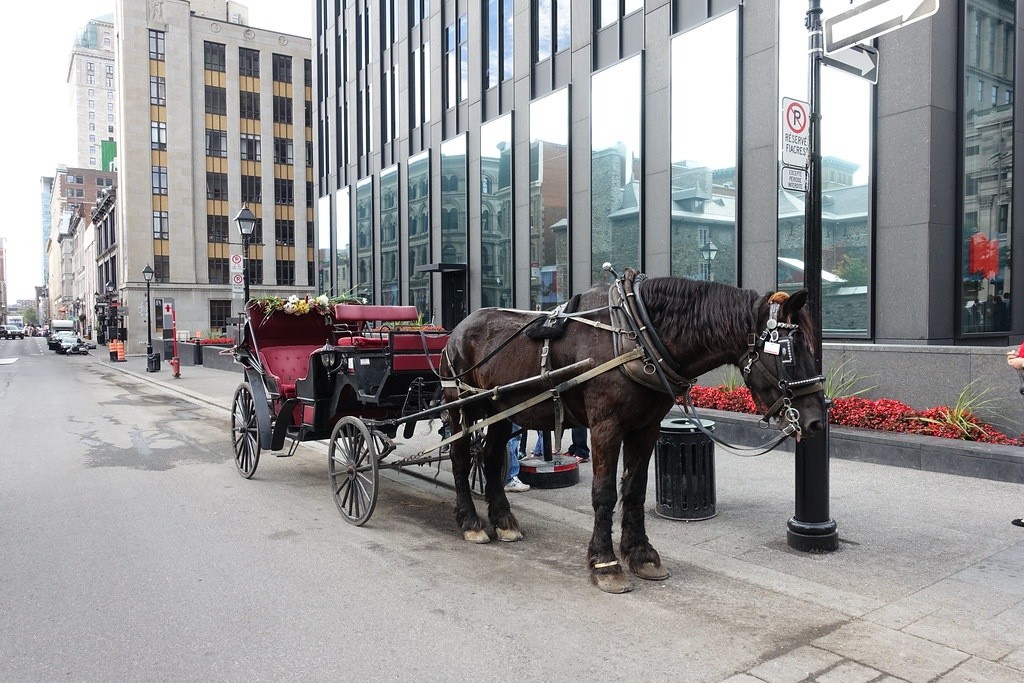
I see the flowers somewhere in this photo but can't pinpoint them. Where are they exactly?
[283,293,330,315]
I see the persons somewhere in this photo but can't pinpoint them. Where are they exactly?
[962,292,1009,331]
[503,422,590,492]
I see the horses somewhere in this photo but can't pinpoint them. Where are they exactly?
[440,278,824,594]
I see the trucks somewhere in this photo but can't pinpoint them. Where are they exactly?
[6,315,24,330]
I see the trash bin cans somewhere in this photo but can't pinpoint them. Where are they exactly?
[152,353,160,370]
[655,419,717,522]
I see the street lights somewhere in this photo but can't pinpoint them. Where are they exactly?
[141,263,156,373]
[232,200,257,401]
[700,239,719,285]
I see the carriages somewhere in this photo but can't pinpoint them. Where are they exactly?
[216,259,829,594]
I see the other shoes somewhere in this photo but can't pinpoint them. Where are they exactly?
[517,451,526,460]
[574,454,589,462]
[1012,518,1024,526]
[564,451,573,456]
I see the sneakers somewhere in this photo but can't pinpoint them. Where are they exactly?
[504,476,529,491]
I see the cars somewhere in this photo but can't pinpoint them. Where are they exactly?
[0,324,24,340]
[46,319,88,356]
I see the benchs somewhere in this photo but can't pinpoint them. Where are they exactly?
[336,304,419,351]
[259,345,329,420]
